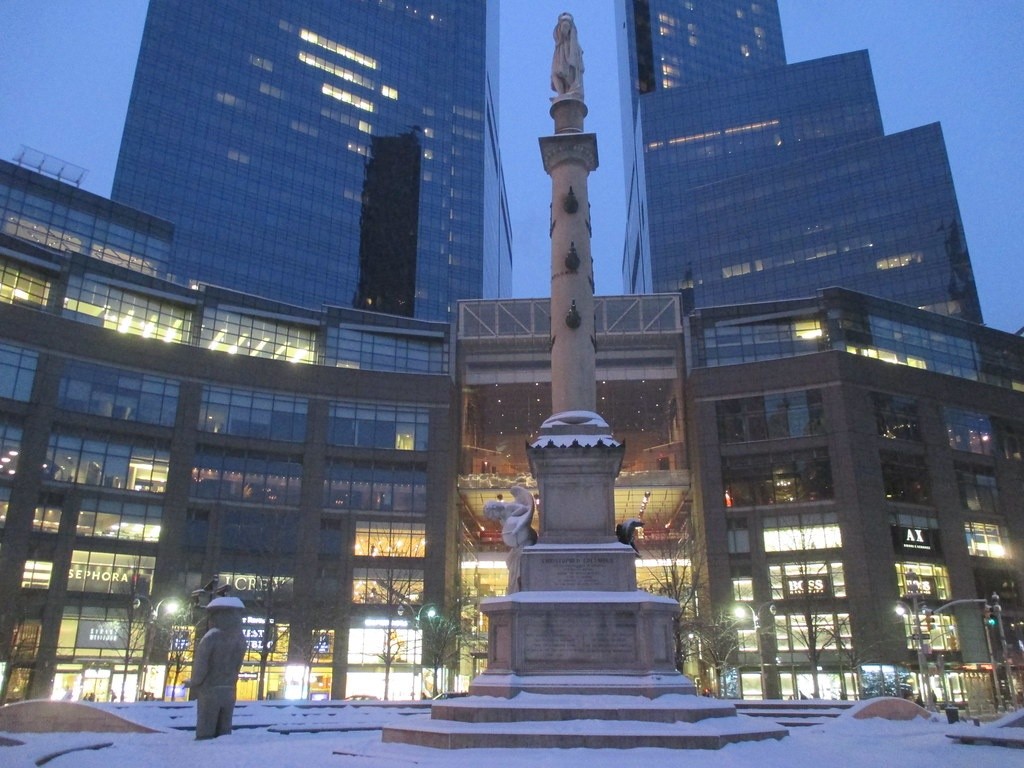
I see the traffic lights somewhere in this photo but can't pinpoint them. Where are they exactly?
[985,604,996,626]
[925,606,936,631]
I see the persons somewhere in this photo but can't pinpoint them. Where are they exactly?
[84,692,95,701]
[484,501,527,526]
[915,696,925,707]
[182,596,246,739]
[150,668,159,678]
[66,686,72,699]
[109,690,117,702]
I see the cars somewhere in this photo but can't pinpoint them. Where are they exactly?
[343,695,378,700]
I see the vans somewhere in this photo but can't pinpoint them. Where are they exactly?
[433,693,467,701]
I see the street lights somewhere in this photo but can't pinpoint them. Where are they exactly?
[133,594,179,702]
[895,601,920,701]
[736,599,776,700]
[396,601,435,701]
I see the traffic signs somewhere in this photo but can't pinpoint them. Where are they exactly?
[912,634,930,639]
